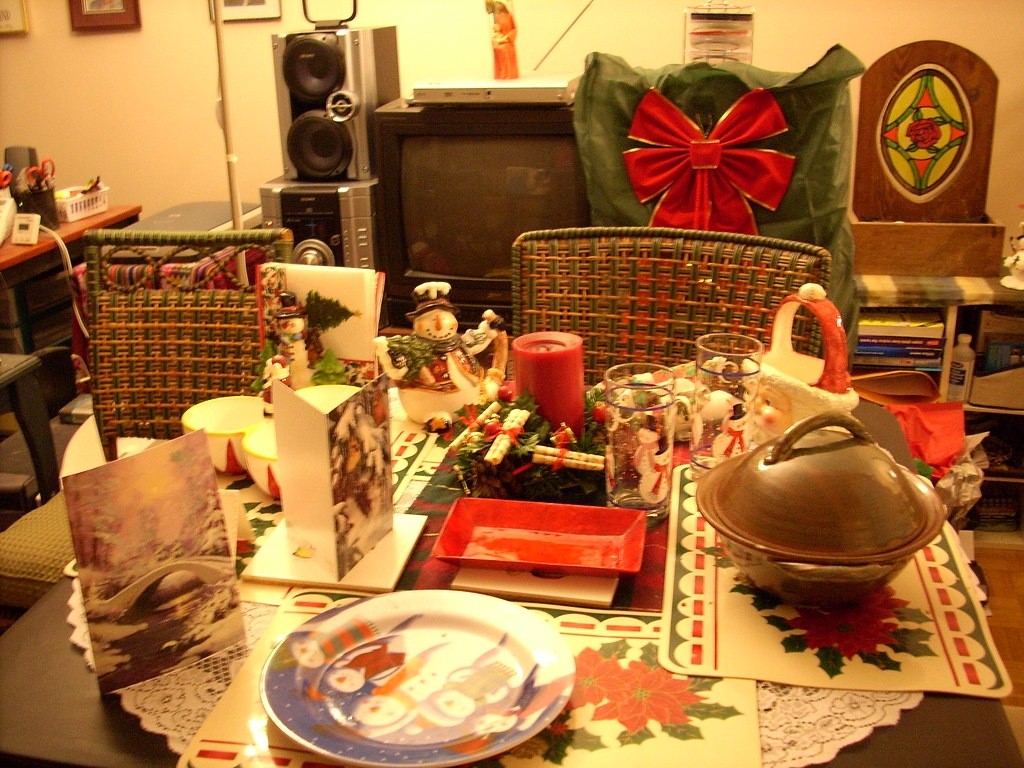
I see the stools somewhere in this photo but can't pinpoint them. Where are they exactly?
[0,352,59,506]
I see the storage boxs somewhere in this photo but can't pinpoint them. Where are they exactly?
[847,206,1007,278]
[56,185,110,222]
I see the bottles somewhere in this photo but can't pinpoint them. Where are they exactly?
[945,333,975,402]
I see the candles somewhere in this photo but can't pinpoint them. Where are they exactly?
[511,332,587,442]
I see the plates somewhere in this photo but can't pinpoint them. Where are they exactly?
[259,589,576,768]
[429,497,646,572]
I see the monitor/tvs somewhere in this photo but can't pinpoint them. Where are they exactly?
[373,92,590,330]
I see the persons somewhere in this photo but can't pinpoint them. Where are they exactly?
[486,1,518,79]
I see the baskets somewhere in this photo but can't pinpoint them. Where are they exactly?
[55,184,110,222]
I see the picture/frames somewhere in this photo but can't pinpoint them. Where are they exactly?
[208,0,282,23]
[69,0,142,33]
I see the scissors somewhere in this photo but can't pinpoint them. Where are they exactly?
[0,169,12,188]
[26,159,56,190]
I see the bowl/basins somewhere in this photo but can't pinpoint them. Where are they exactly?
[695,410,946,606]
[291,384,360,412]
[242,423,282,499]
[181,396,265,476]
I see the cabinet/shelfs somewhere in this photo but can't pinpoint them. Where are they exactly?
[847,272,1024,484]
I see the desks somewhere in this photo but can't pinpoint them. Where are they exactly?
[0,203,142,354]
[0,437,1023,768]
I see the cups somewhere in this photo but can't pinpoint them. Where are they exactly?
[690,333,764,469]
[604,362,677,527]
[13,186,60,231]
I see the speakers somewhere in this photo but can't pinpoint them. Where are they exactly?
[4,146,39,197]
[271,26,400,180]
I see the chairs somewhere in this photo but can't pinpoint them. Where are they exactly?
[509,226,834,384]
[0,228,294,607]
